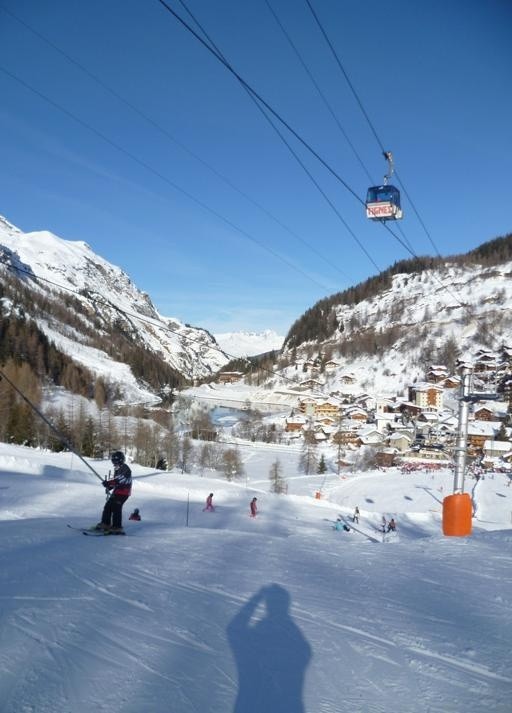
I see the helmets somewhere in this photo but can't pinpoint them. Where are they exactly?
[112,451,124,463]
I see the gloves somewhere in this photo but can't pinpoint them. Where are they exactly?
[102,480,114,490]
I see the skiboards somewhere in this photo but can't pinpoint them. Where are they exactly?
[66,523,127,536]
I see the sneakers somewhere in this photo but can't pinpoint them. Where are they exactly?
[95,522,123,531]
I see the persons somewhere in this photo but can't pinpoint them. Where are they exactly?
[202,492,215,512]
[250,496,258,517]
[395,459,510,482]
[381,515,386,532]
[387,518,396,532]
[127,507,142,521]
[96,449,133,533]
[335,518,350,531]
[353,505,360,524]
